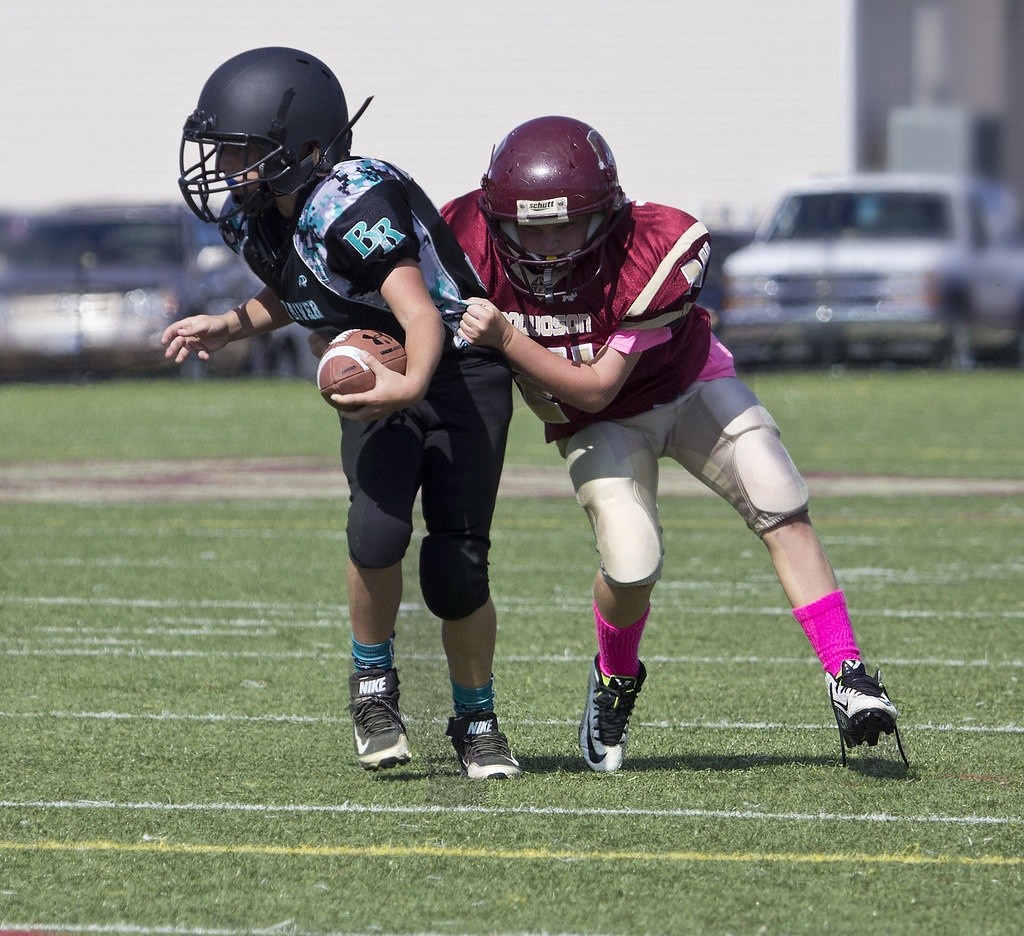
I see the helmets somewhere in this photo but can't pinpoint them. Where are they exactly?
[478,115,621,301]
[182,47,353,194]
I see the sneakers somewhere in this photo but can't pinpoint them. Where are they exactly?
[578,654,648,774]
[346,667,411,770]
[824,657,911,769]
[444,711,522,782]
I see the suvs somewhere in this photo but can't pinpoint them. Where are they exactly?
[720,168,1024,371]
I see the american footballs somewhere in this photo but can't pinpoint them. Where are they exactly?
[317,328,407,412]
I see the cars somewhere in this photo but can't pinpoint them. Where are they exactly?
[1,199,312,382]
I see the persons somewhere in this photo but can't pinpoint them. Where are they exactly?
[159,45,523,781]
[437,112,898,775]
